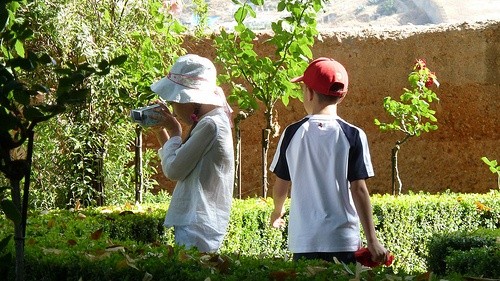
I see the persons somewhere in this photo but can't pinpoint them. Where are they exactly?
[145,53,237,255]
[268,57,386,278]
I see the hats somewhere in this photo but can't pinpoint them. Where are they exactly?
[150,54,225,107]
[290,57,349,96]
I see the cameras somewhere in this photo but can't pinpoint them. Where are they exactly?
[131,99,166,127]
